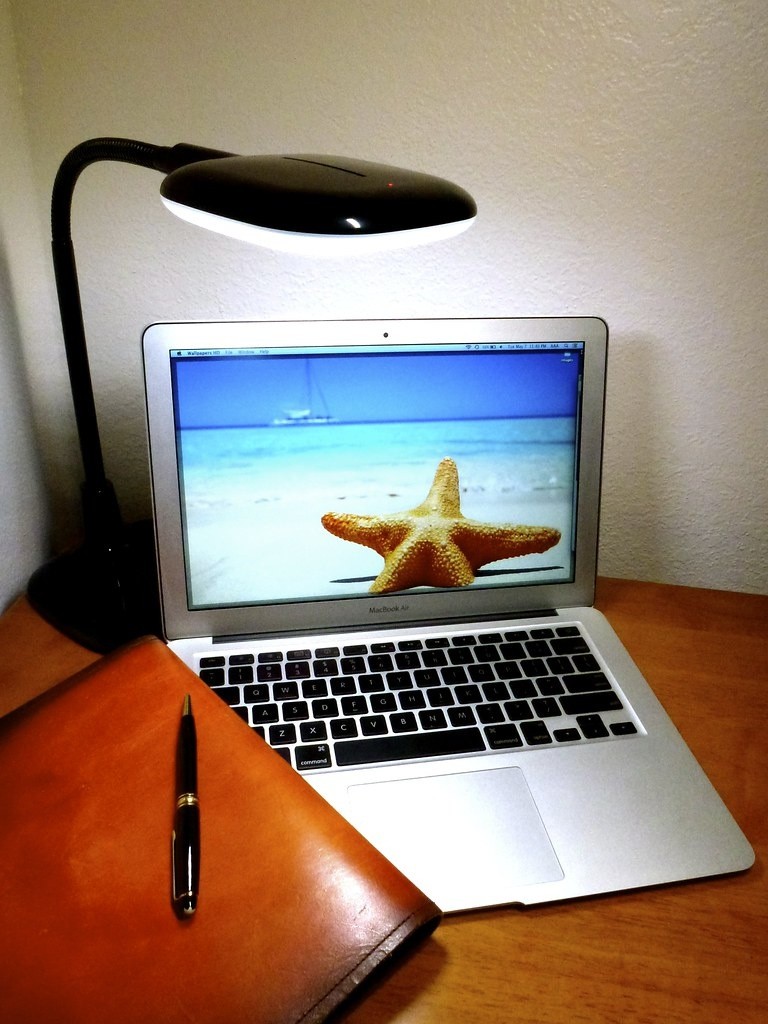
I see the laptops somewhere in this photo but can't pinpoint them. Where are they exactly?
[140,316,754,918]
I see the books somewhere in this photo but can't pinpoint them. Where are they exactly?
[0,634,443,1024]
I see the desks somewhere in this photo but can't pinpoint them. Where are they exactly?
[0,575,768,1024]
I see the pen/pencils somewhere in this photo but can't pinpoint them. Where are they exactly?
[168,694,206,924]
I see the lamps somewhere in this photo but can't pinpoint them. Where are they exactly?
[23,135,480,655]
